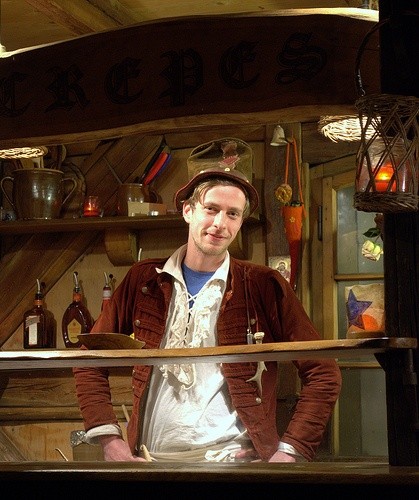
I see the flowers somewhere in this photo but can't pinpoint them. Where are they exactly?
[275,184,302,207]
[361,214,384,262]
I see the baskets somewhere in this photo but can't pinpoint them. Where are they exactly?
[353,9,419,212]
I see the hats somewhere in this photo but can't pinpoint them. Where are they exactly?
[175,137,260,218]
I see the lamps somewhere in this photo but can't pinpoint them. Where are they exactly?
[270,119,288,147]
[354,93,419,211]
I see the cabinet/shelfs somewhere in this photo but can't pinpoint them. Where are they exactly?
[0,211,259,350]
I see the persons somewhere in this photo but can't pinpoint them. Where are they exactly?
[72,137,343,463]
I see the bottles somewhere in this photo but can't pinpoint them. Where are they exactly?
[23,279,56,349]
[62,273,94,348]
[101,272,112,311]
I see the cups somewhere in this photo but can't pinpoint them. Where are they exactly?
[128,184,150,218]
[84,196,104,216]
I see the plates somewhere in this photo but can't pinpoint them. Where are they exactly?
[76,333,145,350]
